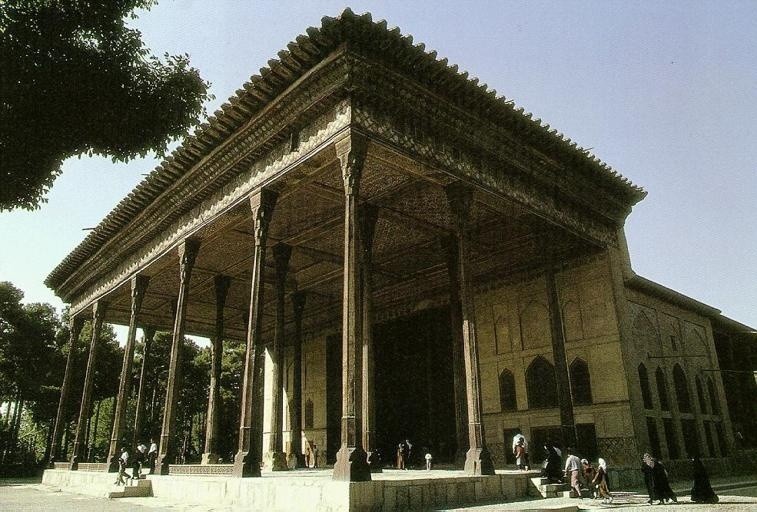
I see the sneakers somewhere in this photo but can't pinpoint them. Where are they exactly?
[647,498,677,504]
[578,494,613,503]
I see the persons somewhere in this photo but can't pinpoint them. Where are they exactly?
[509,429,614,504]
[113,435,158,487]
[313,445,319,468]
[641,450,720,504]
[309,447,316,468]
[306,448,310,468]
[391,438,436,470]
